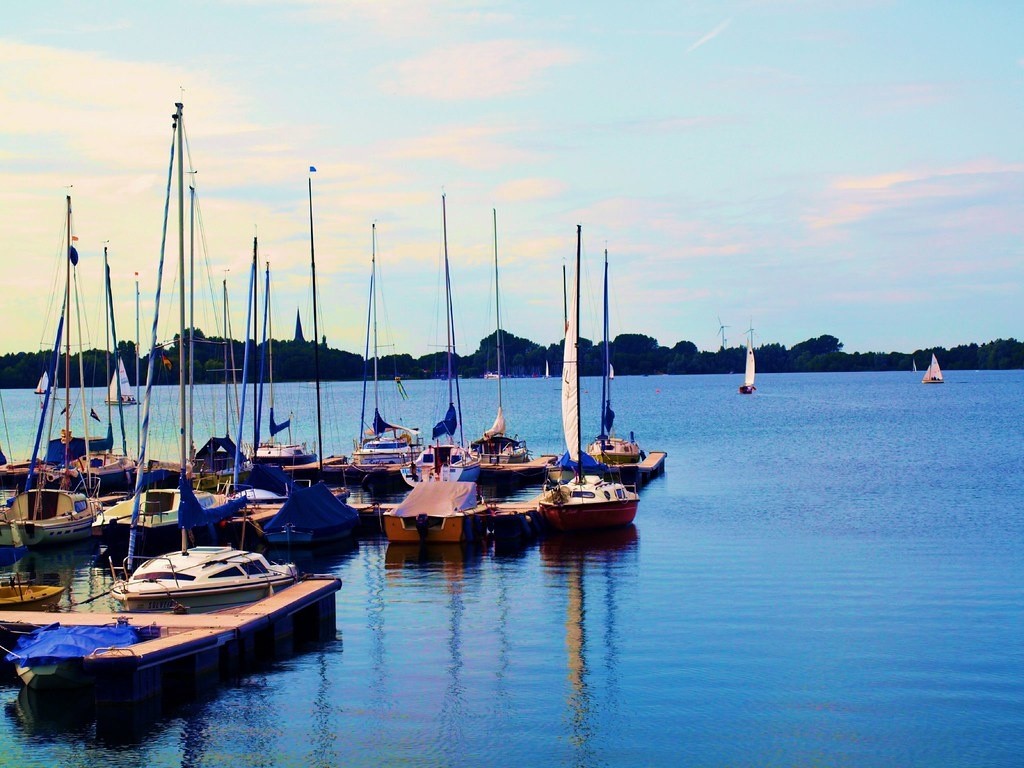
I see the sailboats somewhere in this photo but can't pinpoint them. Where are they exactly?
[921,353,946,384]
[35,371,50,397]
[0,92,673,723]
[738,338,759,394]
[103,356,140,407]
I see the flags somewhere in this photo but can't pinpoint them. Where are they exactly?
[161,350,171,369]
